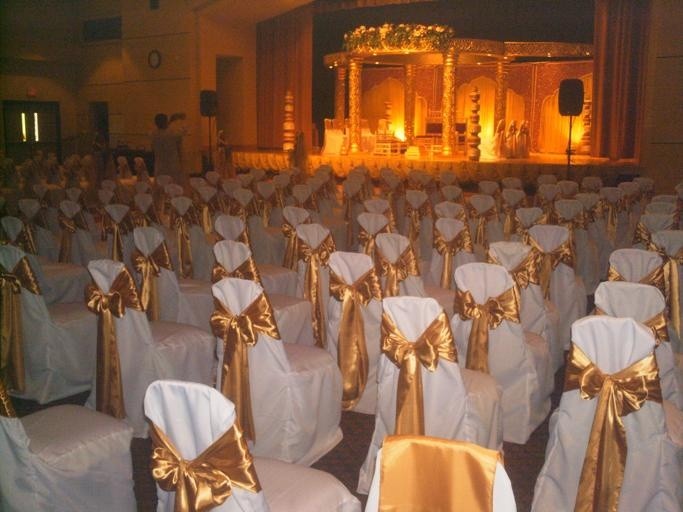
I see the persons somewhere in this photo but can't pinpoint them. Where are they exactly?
[505,119,519,159]
[493,119,507,158]
[151,112,185,177]
[517,119,533,158]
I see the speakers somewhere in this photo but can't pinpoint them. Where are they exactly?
[199,90,218,116]
[558,78,586,117]
